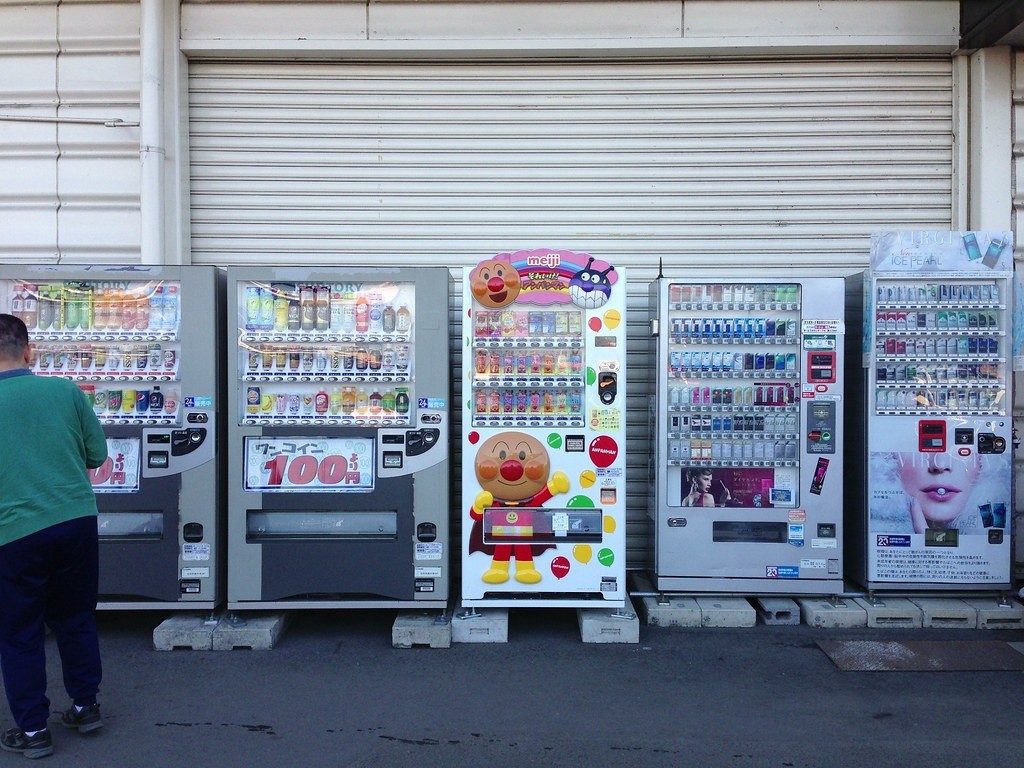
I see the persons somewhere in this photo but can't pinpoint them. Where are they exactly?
[682,467,732,508]
[890,452,982,535]
[0,313,108,759]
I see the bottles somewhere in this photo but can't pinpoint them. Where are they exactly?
[11,284,410,416]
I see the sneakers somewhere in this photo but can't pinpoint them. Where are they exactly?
[0,701,104,759]
